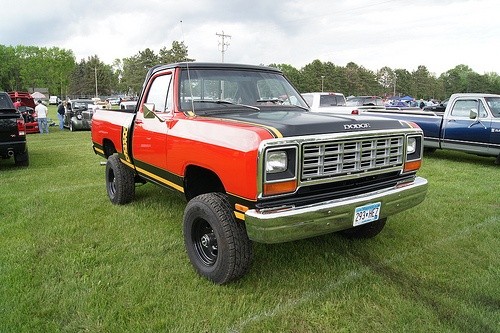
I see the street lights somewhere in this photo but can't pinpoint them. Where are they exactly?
[321,76,325,93]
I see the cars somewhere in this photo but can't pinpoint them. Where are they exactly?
[0,90,31,165]
[345,94,384,106]
[7,91,40,134]
[61,99,100,132]
[90,97,138,112]
[423,98,450,112]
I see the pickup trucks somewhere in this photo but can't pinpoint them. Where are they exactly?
[91,63,429,283]
[351,93,500,163]
[282,92,386,115]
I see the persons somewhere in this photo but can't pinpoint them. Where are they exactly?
[119,98,123,105]
[13,99,22,109]
[382,97,442,111]
[35,100,50,136]
[130,96,137,101]
[57,101,67,131]
[105,99,111,110]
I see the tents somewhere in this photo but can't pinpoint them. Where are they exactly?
[390,95,414,101]
[31,92,46,99]
